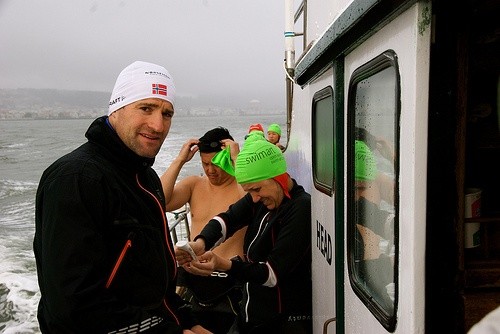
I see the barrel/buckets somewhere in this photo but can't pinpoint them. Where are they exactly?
[463,188,482,249]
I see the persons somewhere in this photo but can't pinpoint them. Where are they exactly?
[33,61,213,334]
[174,135,313,334]
[267,124,281,147]
[355,128,395,312]
[160,127,248,334]
[249,124,264,133]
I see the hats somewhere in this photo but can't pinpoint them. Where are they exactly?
[235,139,287,183]
[108,61,176,116]
[355,140,377,183]
[268,124,281,137]
[249,124,263,132]
